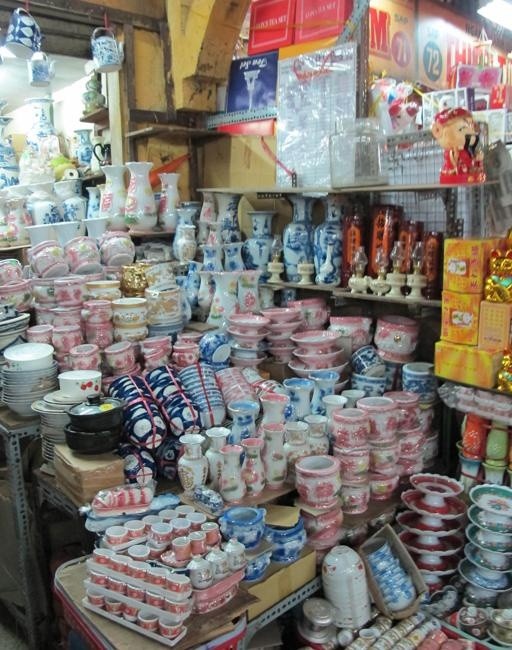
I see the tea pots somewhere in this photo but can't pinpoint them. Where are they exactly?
[91,27,127,73]
[25,51,57,88]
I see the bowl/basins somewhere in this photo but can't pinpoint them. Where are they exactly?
[57,369,103,400]
[3,342,55,371]
[321,539,416,629]
[108,364,261,491]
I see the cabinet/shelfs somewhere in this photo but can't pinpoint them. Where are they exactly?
[193,186,457,309]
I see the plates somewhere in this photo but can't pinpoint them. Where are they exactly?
[286,358,350,377]
[2,361,59,418]
[226,308,347,366]
[30,389,104,466]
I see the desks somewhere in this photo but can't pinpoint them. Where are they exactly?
[0,407,94,650]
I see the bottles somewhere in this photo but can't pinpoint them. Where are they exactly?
[218,443,247,503]
[261,368,365,492]
[240,437,265,495]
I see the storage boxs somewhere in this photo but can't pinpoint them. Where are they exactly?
[238,544,317,623]
[435,235,512,390]
[52,443,125,507]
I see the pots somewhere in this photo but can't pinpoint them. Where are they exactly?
[62,394,129,454]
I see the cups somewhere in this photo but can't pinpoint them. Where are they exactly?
[458,606,490,638]
[336,612,446,650]
[87,503,246,639]
[490,608,512,644]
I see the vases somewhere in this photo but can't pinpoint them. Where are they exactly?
[27,162,345,329]
[0,97,92,190]
[174,371,340,505]
